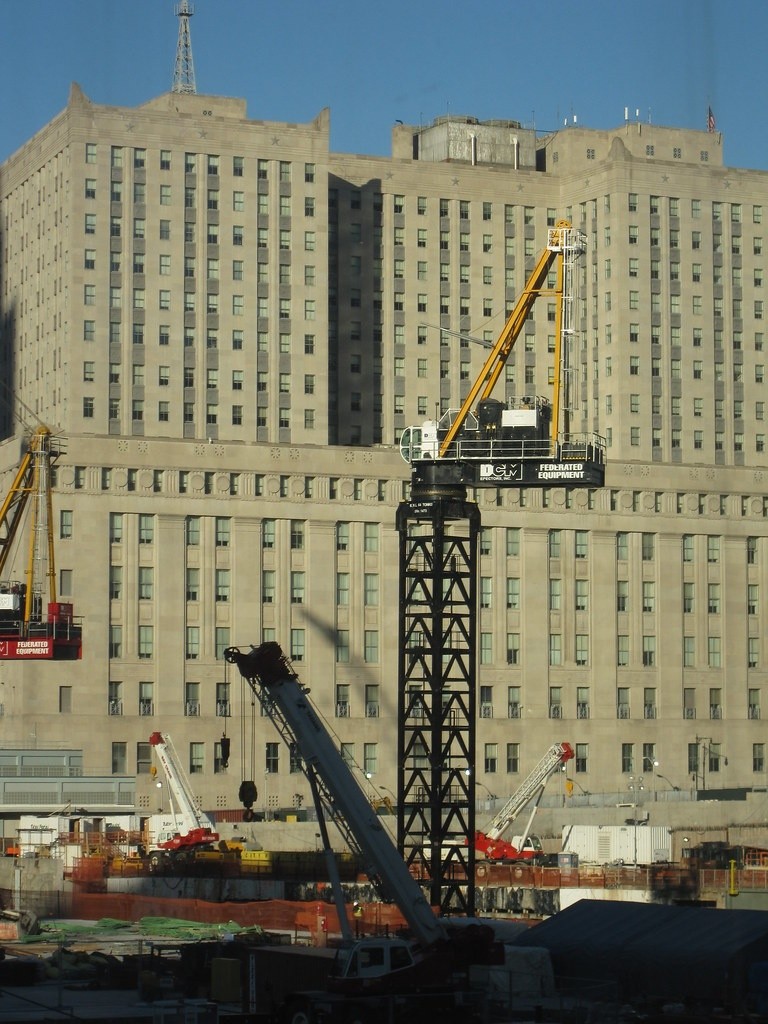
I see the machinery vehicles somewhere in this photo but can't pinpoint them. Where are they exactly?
[464,741,575,867]
[149,731,221,867]
[220,641,504,1024]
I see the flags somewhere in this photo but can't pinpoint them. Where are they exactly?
[706,106,715,132]
